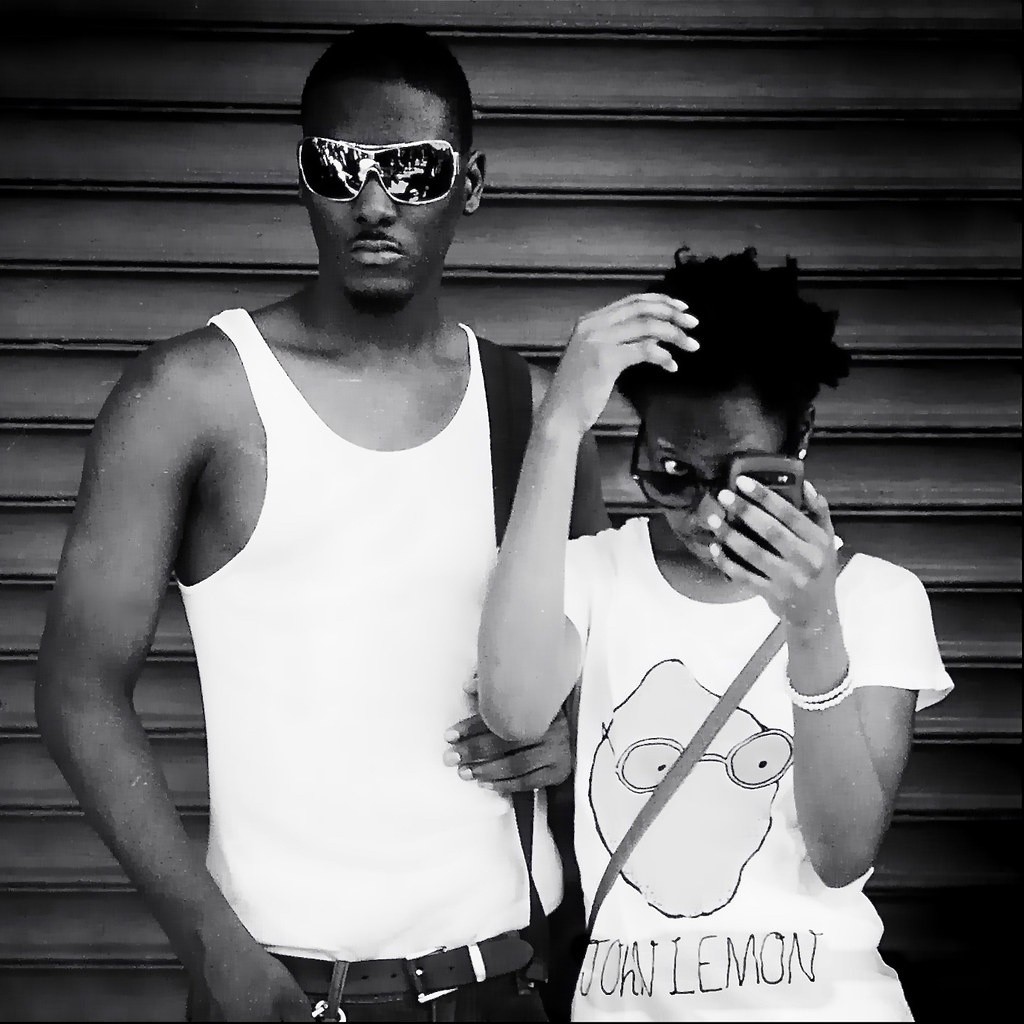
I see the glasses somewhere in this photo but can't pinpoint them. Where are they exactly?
[296,136,471,206]
[628,413,806,511]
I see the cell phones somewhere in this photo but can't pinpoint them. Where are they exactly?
[725,453,805,581]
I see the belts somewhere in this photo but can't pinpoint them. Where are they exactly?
[268,930,545,1004]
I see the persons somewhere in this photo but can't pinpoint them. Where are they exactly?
[475,249,959,1023]
[30,20,610,1023]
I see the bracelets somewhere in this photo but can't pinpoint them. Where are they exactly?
[785,647,855,713]
